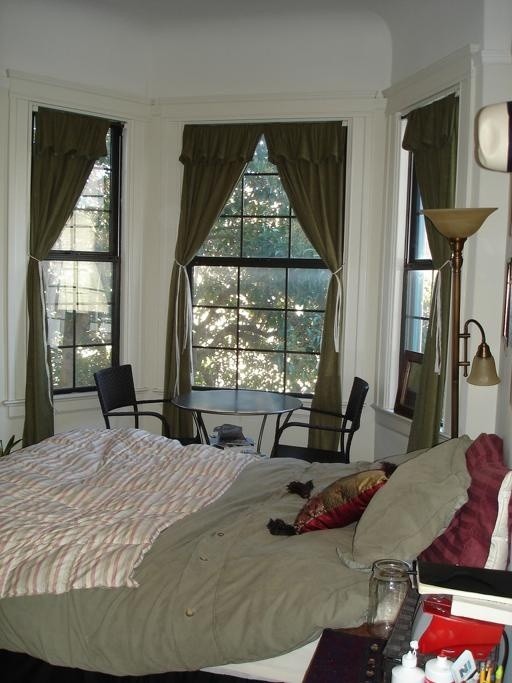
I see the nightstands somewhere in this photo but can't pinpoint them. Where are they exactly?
[304,627,387,683]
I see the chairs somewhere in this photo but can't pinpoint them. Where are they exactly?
[91,364,370,464]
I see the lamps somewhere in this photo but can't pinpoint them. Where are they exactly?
[420,207,501,439]
[475,102,511,174]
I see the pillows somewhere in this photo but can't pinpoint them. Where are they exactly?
[267,433,511,574]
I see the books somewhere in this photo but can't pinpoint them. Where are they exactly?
[416,560,512,605]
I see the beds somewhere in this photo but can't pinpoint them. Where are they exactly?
[1,426,430,682]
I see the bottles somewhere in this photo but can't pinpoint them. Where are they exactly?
[367,558,412,640]
